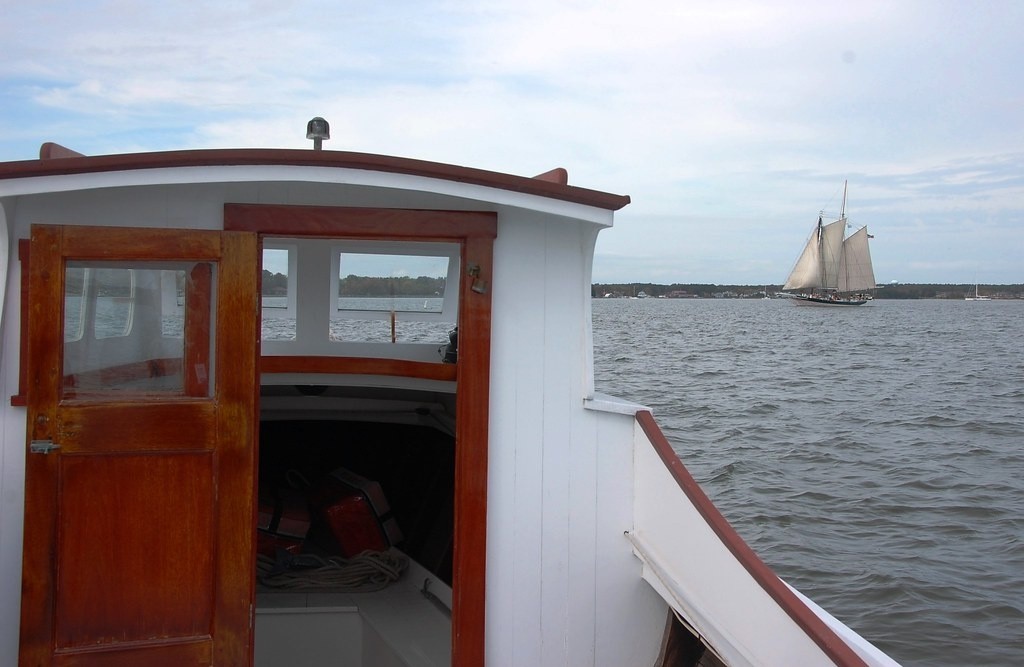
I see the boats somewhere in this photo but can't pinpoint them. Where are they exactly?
[964,284,991,301]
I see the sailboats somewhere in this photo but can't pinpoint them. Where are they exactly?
[782,178,877,305]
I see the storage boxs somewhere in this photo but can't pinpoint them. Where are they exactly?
[304,466,404,558]
[257,485,312,555]
